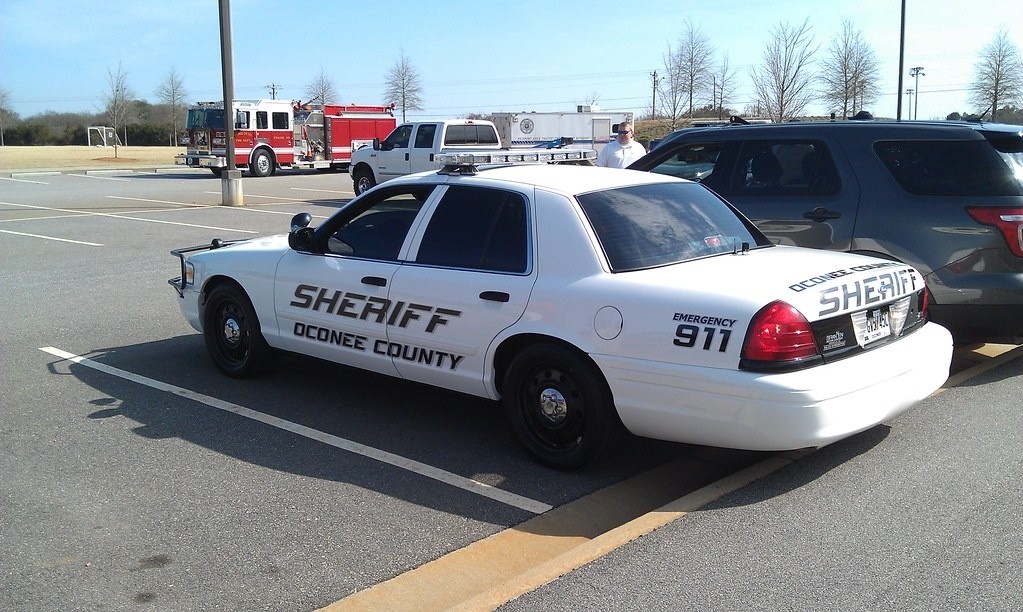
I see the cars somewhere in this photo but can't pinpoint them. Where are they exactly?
[166,143,956,472]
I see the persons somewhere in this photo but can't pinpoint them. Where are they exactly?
[595,122,647,169]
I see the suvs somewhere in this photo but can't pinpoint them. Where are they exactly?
[348,117,502,199]
[615,117,1022,353]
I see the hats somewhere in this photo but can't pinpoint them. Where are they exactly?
[730,116,750,125]
[847,111,873,121]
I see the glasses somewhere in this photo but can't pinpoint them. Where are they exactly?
[617,130,631,135]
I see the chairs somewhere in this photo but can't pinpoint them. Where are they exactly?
[747,153,784,195]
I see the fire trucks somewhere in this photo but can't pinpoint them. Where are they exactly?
[183,97,399,178]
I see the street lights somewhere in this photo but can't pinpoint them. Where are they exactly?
[905,88,913,119]
[910,66,926,121]
